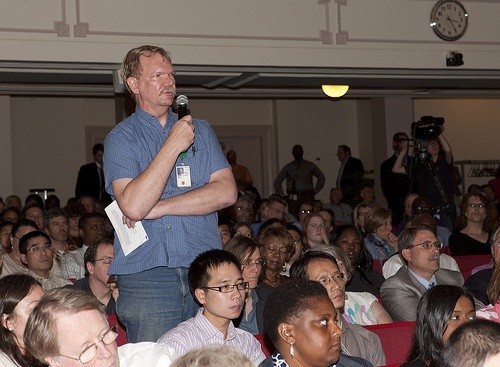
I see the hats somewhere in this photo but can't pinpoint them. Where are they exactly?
[393,132,413,142]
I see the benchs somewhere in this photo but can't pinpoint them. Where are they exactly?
[108,247,500,367]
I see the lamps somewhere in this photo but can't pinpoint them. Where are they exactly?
[321,84,349,99]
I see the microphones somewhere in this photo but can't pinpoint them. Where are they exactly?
[176,95,189,159]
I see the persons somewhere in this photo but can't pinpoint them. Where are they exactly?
[226,150,252,191]
[0,184,500,367]
[336,145,363,197]
[407,124,457,217]
[274,145,326,219]
[178,168,182,175]
[104,46,238,343]
[381,131,415,226]
[75,144,111,201]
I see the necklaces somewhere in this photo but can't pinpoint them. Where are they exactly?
[265,274,278,288]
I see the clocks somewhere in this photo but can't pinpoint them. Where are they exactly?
[430,0,468,41]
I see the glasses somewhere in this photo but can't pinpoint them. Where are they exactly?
[468,203,485,209]
[409,241,444,250]
[299,209,313,215]
[201,282,250,293]
[415,207,433,214]
[241,258,266,268]
[27,244,53,254]
[55,325,119,365]
